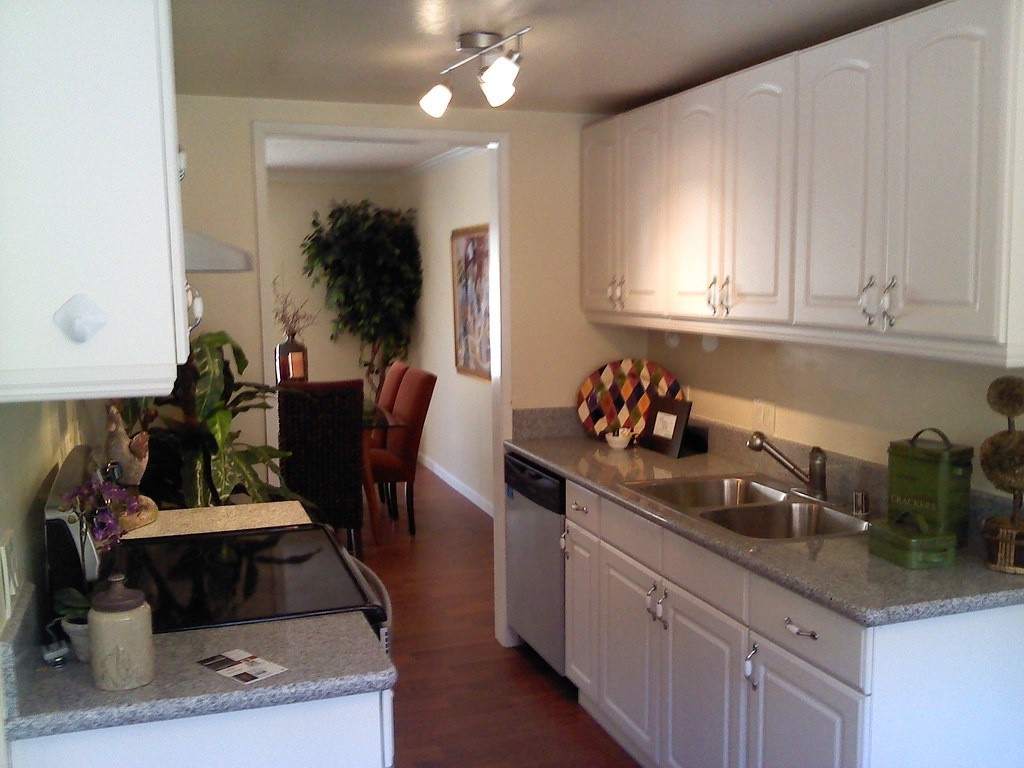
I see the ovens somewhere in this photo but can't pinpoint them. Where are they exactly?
[352,556,395,768]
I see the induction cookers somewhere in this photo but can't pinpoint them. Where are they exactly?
[45,444,388,635]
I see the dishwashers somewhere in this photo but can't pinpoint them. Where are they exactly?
[504,450,577,701]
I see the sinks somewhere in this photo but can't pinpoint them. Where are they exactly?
[670,502,886,543]
[616,472,813,510]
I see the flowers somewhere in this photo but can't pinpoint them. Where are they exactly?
[53,483,146,621]
[272,276,320,337]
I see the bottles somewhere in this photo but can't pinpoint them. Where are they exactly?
[88,574,154,691]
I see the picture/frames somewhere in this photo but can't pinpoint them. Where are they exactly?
[449,227,492,378]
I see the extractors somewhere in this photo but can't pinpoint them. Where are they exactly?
[184,229,249,271]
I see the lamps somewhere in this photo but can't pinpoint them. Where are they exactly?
[419,21,533,117]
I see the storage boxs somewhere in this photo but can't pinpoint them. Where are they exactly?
[867,514,956,572]
[885,428,974,548]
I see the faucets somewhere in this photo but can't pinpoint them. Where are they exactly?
[745,431,827,491]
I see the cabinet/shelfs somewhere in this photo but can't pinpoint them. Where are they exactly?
[577,0,1024,369]
[0,0,201,403]
[564,484,1024,768]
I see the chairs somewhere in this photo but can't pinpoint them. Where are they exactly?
[364,360,408,516]
[364,369,436,534]
[279,378,367,558]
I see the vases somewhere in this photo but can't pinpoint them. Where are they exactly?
[275,332,307,383]
[58,613,90,663]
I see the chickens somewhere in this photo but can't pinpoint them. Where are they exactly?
[104,403,151,488]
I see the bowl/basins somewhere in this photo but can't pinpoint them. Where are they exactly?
[606,433,631,449]
